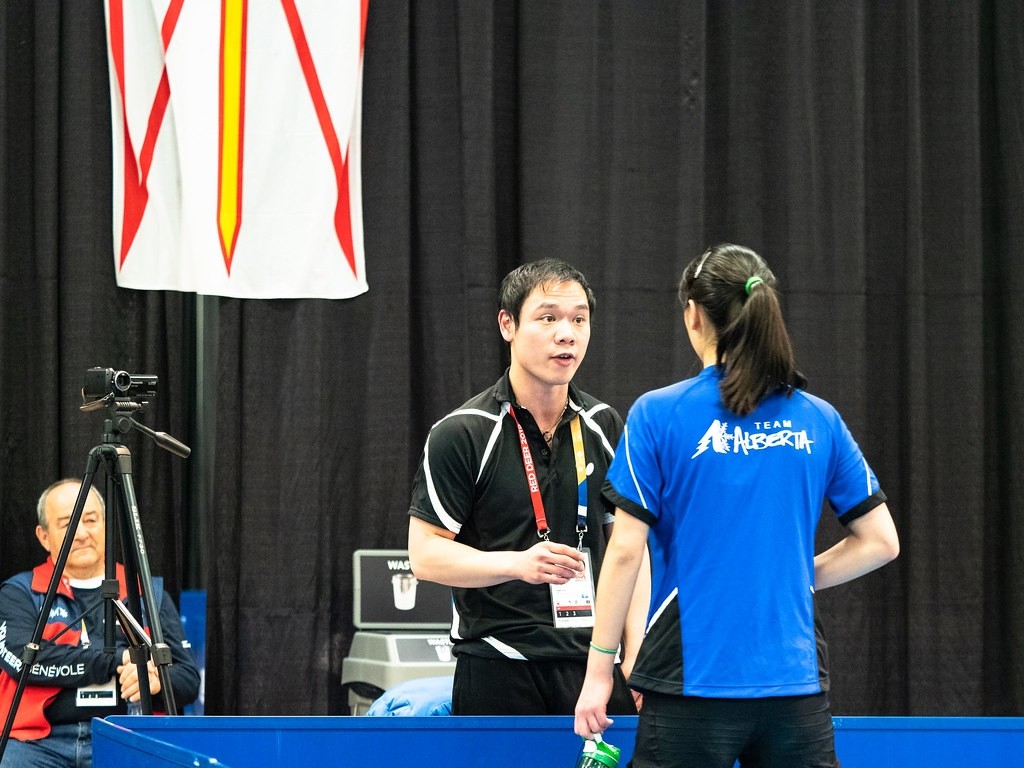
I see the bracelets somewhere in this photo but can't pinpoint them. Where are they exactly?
[590,641,617,656]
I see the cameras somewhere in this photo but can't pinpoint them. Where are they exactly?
[86,368,159,402]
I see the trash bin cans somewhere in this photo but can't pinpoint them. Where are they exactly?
[341,630,458,717]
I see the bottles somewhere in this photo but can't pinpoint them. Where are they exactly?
[125,697,142,716]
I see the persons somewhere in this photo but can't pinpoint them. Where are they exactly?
[573,242,900,768]
[0,477,203,767]
[405,258,652,718]
[575,560,585,579]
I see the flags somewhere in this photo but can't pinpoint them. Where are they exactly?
[105,1,371,301]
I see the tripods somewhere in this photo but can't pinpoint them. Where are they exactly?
[0,401,191,762]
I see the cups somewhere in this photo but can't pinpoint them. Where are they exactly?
[576,733,621,768]
[392,574,417,610]
